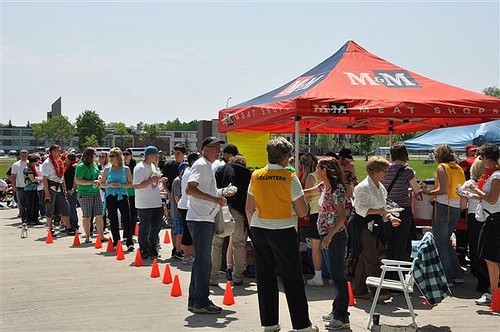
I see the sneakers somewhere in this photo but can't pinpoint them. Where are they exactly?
[208,284,226,294]
[323,313,334,321]
[188,300,222,313]
[325,318,350,330]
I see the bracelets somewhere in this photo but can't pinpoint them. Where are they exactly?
[307,202,310,215]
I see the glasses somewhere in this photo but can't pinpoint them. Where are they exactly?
[122,153,130,156]
[206,145,221,150]
[108,155,118,158]
[479,155,483,160]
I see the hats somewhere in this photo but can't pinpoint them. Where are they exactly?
[339,148,353,160]
[178,162,189,172]
[145,146,158,155]
[202,136,226,150]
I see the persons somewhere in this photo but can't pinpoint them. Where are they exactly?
[0,144,423,294]
[303,157,351,330]
[454,135,500,305]
[132,146,168,265]
[42,145,80,237]
[246,137,319,332]
[185,137,237,313]
[351,154,400,302]
[74,147,109,243]
[420,144,466,287]
[100,147,134,249]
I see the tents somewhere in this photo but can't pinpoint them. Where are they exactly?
[219,40,500,234]
[402,118,500,150]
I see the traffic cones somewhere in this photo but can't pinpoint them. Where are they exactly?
[162,264,173,284]
[164,231,170,243]
[347,281,354,305]
[490,288,499,312]
[46,231,53,244]
[135,250,143,267]
[107,237,114,252]
[151,258,160,278]
[116,240,125,260]
[171,275,182,297]
[222,281,235,305]
[95,234,103,248]
[73,232,80,246]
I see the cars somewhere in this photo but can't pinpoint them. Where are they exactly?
[9,150,17,156]
[0,150,6,156]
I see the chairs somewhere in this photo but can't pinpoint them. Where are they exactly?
[366,232,452,332]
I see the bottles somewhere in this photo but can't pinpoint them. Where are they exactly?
[222,205,231,223]
[156,167,160,177]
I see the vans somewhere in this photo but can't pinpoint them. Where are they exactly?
[95,147,111,156]
[126,148,145,156]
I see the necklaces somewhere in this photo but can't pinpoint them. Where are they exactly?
[49,154,63,178]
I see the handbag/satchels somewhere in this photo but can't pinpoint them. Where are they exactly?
[412,192,433,220]
[215,209,225,235]
[474,199,486,222]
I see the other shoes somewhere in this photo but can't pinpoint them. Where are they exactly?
[447,277,495,306]
[264,325,282,332]
[16,212,125,251]
[141,249,150,258]
[225,271,233,280]
[233,286,254,295]
[303,265,393,303]
[292,325,319,332]
[128,245,135,251]
[182,255,193,264]
[171,249,185,260]
[150,254,157,263]
[232,280,250,287]
